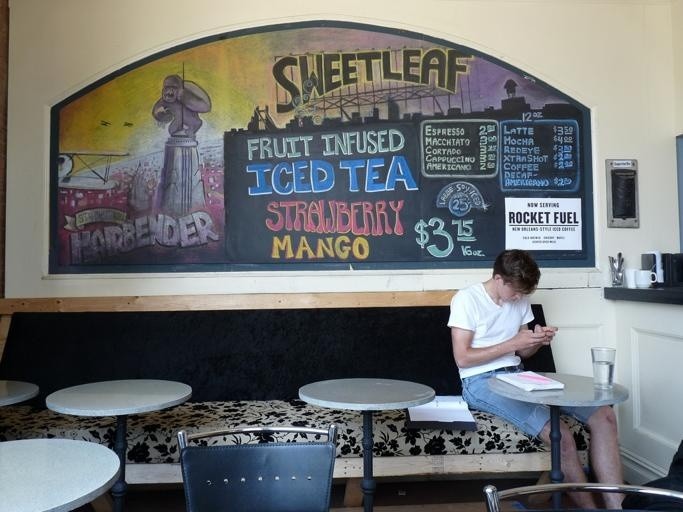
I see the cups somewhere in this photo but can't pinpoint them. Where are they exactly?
[591,347,616,390]
[624,268,638,287]
[609,268,624,286]
[634,270,657,287]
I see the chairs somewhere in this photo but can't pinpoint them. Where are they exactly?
[176,423,338,512]
[480,484,682,512]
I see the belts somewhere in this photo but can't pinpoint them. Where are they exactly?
[486,366,520,372]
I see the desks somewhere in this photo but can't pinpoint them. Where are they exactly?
[0,380,39,407]
[45,379,192,512]
[485,373,629,512]
[298,378,435,512]
[0,438,122,512]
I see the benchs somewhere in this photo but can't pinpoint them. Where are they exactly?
[0,305,590,512]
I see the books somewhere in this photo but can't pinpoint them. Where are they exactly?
[496,370,564,392]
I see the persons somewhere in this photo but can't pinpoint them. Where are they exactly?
[447,249,626,511]
